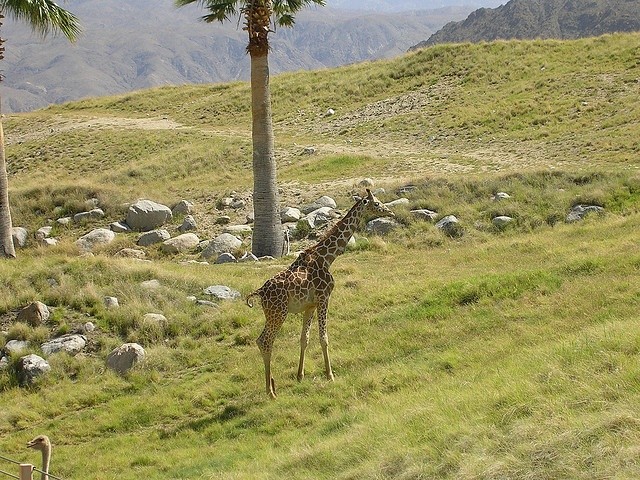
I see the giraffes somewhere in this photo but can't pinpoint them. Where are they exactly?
[246,189,396,400]
